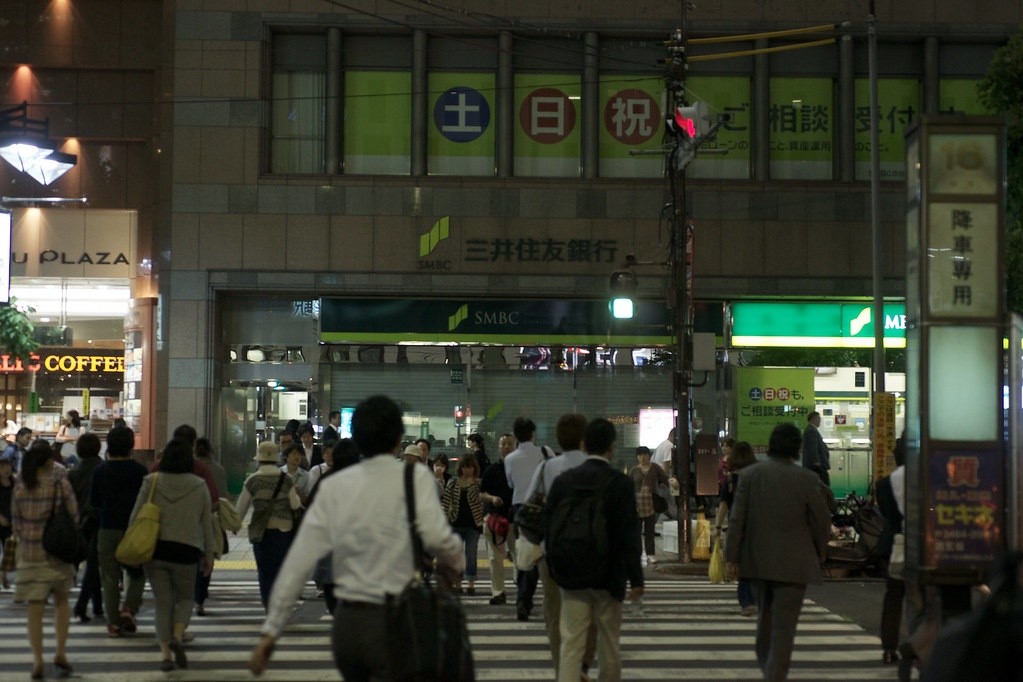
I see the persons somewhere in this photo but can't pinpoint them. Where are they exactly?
[0,396,759,682]
[724,424,831,682]
[802,412,841,516]
[873,430,1023,682]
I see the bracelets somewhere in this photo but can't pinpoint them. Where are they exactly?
[715,525,722,529]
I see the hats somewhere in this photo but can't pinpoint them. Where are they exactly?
[252,440,281,462]
[404,444,424,461]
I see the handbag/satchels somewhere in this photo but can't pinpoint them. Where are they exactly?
[383,461,476,681]
[487,515,510,547]
[246,508,269,543]
[652,493,668,513]
[113,472,161,566]
[708,537,725,584]
[41,481,91,563]
[514,489,547,545]
[51,442,62,453]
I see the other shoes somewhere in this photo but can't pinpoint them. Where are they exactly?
[883,644,915,681]
[195,607,205,616]
[647,555,658,563]
[161,640,188,673]
[73,606,104,623]
[31,666,44,679]
[181,632,194,642]
[490,593,507,605]
[54,656,70,670]
[517,602,529,622]
[109,606,136,637]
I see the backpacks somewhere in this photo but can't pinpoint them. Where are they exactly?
[544,466,619,591]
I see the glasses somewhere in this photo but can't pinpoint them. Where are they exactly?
[282,440,291,445]
